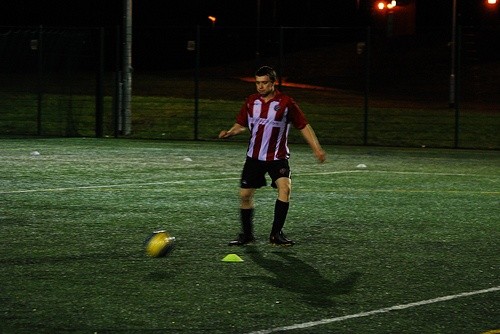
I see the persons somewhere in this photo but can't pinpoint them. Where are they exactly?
[218,65,326,247]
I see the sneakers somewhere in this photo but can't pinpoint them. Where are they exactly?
[269,233,295,248]
[228,234,255,247]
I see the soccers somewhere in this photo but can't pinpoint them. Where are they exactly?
[145,231,172,257]
